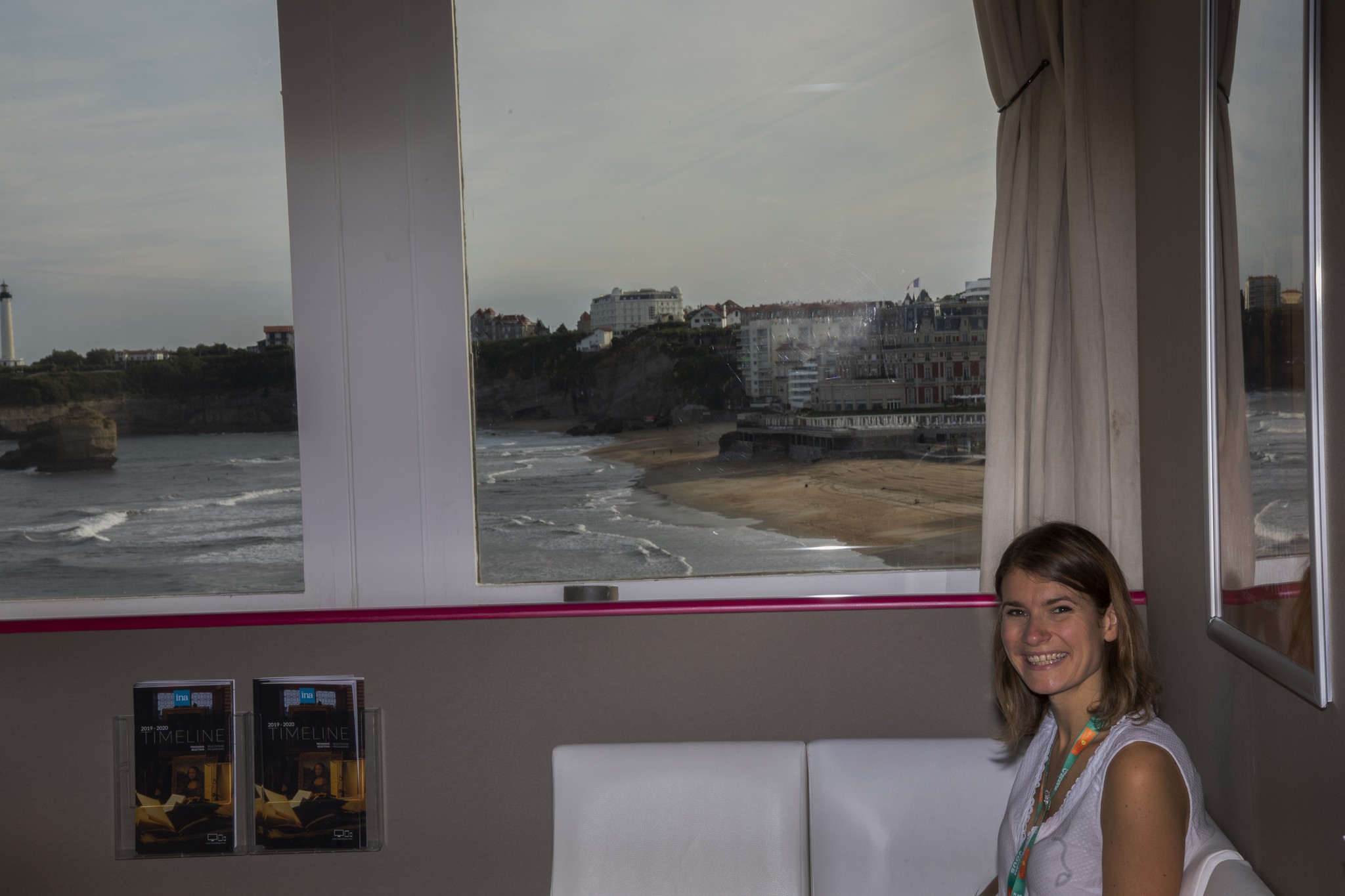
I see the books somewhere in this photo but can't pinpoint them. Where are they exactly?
[131,674,368,858]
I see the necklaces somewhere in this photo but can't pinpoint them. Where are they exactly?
[1043,733,1100,812]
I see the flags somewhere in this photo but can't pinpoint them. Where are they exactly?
[907,277,919,291]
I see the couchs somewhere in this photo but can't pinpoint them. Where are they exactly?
[551,740,1281,896]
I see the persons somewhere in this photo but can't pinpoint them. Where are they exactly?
[980,522,1200,896]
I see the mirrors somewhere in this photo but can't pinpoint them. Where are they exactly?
[1198,0,1330,711]
